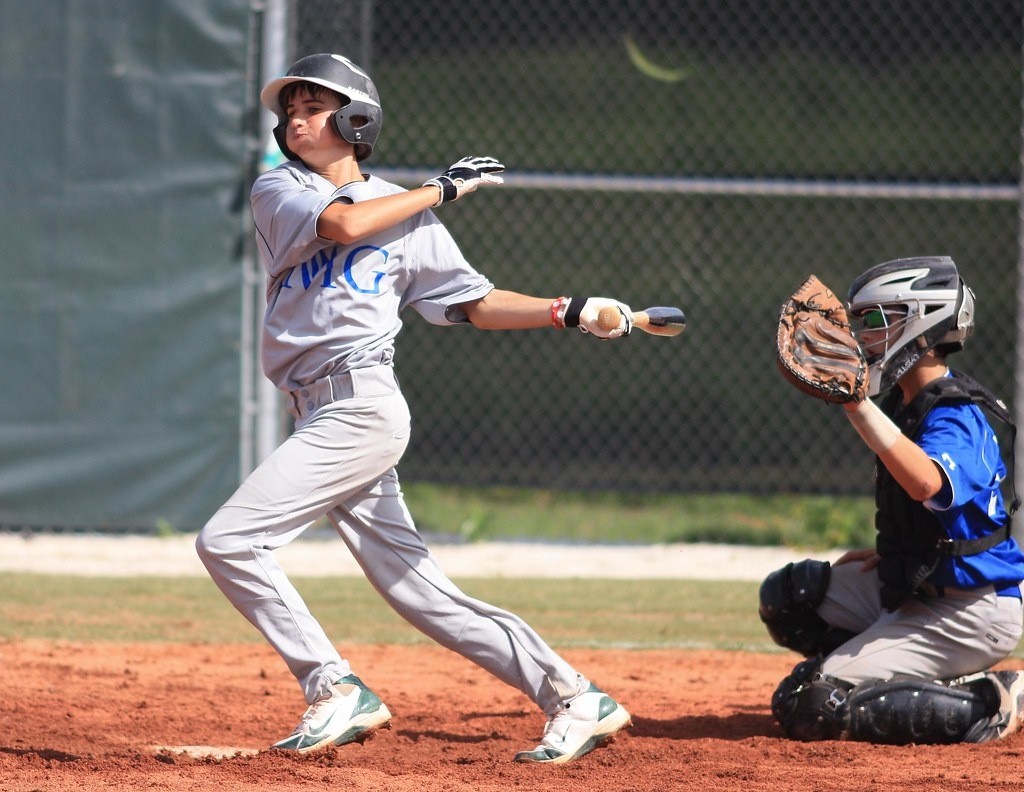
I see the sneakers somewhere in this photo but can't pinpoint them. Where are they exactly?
[962,670,1024,744]
[515,673,632,767]
[270,673,393,756]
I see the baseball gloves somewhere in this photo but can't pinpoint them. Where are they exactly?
[772,272,872,406]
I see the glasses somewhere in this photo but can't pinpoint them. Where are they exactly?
[857,312,892,331]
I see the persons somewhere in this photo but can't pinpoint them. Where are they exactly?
[193,54,635,762]
[756,256,1023,744]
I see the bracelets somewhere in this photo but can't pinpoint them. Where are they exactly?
[551,297,565,329]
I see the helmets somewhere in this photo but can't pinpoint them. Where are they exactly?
[259,52,381,163]
[845,256,974,397]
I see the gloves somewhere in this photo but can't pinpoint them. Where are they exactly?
[423,155,506,208]
[551,296,636,341]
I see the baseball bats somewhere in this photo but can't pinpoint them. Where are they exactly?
[597,305,686,338]
